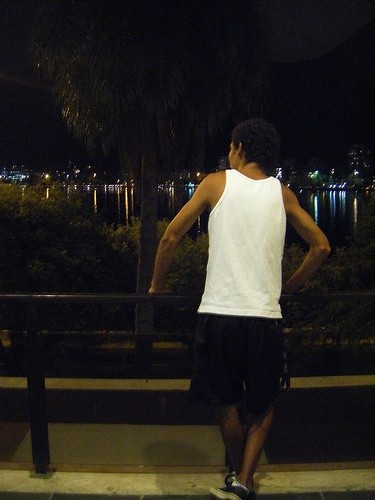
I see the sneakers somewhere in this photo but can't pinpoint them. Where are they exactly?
[225,474,256,499]
[210,487,249,500]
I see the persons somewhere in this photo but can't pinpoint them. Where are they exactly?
[148,119,331,500]
